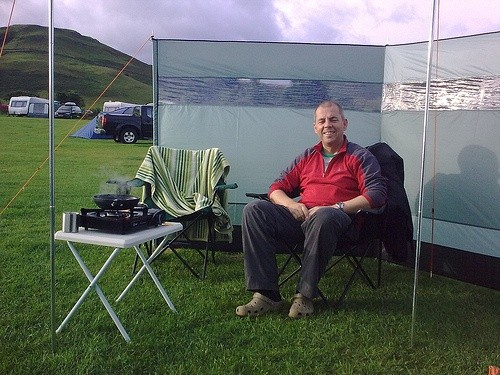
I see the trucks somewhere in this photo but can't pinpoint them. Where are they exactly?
[9,97,60,117]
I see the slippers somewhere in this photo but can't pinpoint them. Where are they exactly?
[236,292,283,317]
[288,293,315,317]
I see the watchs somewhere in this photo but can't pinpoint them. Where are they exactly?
[336,202,344,210]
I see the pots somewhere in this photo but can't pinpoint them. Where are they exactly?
[93,194,140,210]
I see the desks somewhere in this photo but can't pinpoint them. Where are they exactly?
[53,220,184,347]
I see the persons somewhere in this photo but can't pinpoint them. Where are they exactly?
[235,100,389,320]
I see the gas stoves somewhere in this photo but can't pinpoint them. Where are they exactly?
[79,206,166,235]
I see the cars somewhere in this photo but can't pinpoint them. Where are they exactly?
[54,105,82,119]
[94,105,153,144]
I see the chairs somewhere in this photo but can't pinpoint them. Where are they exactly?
[245,142,414,312]
[125,146,238,287]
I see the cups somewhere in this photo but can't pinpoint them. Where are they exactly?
[63,212,79,233]
